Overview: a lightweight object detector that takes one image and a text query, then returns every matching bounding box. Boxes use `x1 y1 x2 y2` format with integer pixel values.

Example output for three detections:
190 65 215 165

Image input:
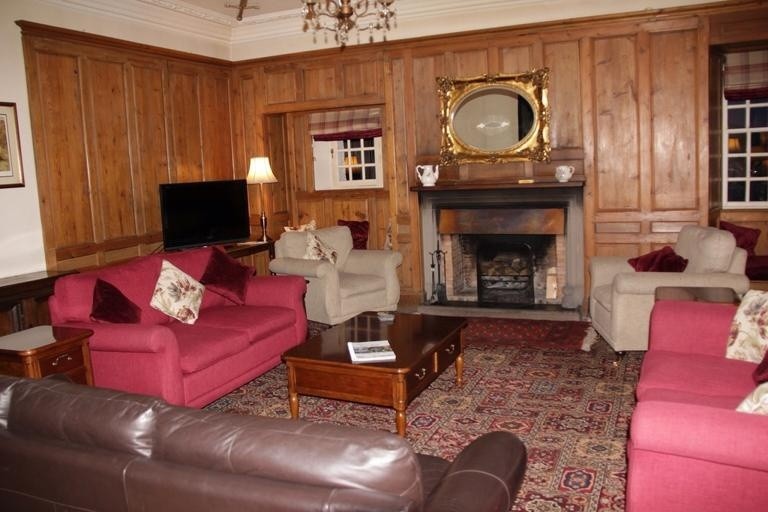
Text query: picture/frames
0 102 25 189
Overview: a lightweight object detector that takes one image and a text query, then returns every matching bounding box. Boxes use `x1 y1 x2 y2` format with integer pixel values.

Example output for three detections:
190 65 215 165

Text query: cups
554 166 576 182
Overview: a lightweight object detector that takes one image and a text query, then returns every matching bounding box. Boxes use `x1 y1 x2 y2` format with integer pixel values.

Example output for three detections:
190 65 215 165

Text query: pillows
54 245 256 324
628 220 762 272
279 219 369 267
726 289 768 416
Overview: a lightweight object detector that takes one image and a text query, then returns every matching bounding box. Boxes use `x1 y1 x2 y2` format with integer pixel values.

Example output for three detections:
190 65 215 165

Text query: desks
0 271 80 332
164 240 276 276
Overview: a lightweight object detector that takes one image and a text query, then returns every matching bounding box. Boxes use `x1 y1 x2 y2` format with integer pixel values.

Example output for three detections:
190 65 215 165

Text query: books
347 340 397 365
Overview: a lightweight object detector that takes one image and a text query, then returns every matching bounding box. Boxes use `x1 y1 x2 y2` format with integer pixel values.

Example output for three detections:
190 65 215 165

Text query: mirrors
435 66 552 166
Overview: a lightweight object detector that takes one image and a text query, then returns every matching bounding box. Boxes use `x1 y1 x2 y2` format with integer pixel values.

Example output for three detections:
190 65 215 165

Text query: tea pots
416 163 440 187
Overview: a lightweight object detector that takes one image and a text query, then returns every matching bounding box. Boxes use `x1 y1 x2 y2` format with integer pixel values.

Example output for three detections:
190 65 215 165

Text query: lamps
300 0 397 50
246 157 278 242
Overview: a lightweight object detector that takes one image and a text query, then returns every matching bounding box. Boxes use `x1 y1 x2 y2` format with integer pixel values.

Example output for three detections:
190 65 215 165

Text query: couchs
0 373 526 512
48 244 309 408
626 301 768 512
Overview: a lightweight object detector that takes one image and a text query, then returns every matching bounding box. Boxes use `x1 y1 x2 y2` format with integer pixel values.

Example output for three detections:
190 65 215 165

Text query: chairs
268 226 403 326
588 225 750 359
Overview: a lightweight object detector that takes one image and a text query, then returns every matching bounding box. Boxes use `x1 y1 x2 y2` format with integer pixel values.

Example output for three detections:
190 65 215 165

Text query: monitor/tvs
159 178 251 251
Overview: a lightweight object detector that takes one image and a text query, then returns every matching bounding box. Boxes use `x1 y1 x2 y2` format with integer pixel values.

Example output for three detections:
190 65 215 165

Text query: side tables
0 325 96 387
655 286 741 304
